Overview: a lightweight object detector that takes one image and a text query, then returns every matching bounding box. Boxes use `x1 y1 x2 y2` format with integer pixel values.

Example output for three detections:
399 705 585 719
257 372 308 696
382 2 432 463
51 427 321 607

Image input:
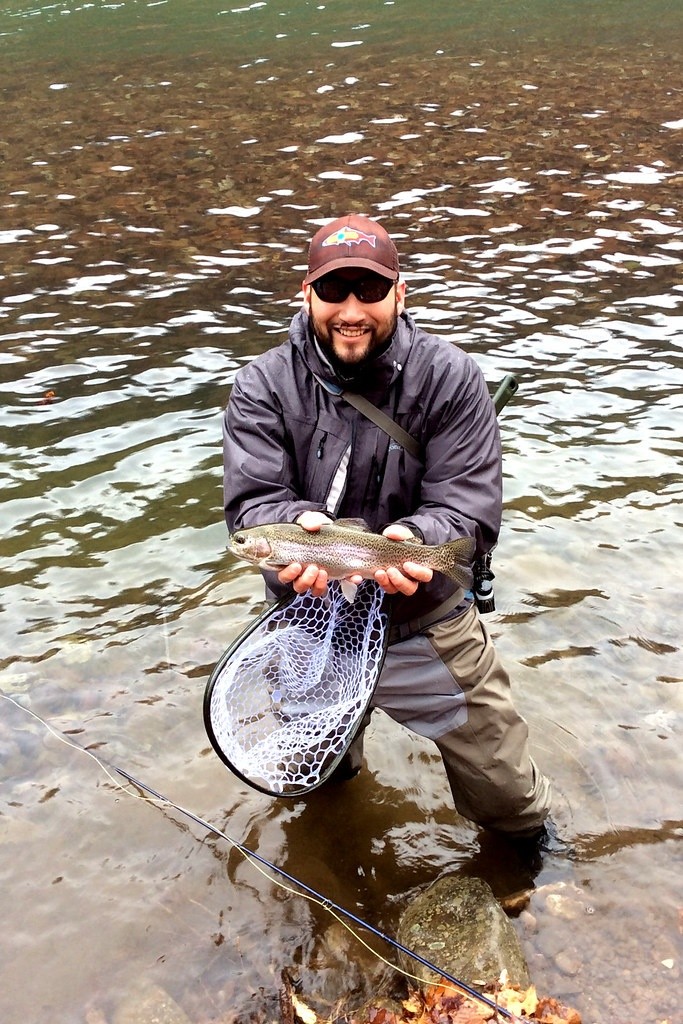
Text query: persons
224 213 575 887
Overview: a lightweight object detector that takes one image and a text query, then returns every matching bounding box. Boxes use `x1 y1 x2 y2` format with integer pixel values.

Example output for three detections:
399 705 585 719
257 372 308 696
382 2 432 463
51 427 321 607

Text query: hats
304 214 399 287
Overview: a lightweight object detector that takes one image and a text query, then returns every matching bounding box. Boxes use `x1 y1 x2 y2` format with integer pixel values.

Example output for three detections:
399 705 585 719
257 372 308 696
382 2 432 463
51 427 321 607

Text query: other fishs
222 517 479 602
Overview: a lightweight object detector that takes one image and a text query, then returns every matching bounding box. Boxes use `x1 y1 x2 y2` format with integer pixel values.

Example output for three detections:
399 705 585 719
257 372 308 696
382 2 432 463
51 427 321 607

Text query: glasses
311 277 394 304
